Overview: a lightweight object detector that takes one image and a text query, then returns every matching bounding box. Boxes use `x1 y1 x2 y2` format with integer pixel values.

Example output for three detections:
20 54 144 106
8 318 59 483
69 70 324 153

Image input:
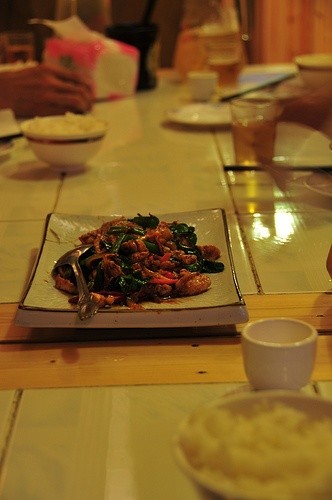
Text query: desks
0 64 332 500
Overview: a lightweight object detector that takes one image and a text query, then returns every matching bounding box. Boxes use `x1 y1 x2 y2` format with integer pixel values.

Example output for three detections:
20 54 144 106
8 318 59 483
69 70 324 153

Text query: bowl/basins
21 115 109 171
171 389 332 500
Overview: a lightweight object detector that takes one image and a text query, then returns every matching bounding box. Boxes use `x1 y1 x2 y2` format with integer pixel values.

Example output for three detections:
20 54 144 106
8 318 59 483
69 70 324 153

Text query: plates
12 206 250 327
266 120 332 169
167 102 248 127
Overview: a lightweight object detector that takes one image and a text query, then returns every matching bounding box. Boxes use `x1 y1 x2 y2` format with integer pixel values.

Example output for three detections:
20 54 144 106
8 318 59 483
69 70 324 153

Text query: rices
28 112 103 134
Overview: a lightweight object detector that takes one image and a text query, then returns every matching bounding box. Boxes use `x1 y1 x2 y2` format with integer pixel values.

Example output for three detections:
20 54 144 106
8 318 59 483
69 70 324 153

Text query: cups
241 317 318 391
294 53 332 83
187 70 218 102
230 97 278 185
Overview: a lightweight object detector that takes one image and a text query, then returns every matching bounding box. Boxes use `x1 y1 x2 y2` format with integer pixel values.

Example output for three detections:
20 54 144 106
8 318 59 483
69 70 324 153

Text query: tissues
26 13 141 102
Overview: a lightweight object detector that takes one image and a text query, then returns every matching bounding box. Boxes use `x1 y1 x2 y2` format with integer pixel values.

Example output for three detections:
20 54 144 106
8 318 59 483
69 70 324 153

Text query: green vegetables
51 212 225 296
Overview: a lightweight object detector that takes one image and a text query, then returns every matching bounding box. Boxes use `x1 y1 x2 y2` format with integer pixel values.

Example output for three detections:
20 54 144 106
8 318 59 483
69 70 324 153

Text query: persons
0 61 93 121
325 246 332 278
